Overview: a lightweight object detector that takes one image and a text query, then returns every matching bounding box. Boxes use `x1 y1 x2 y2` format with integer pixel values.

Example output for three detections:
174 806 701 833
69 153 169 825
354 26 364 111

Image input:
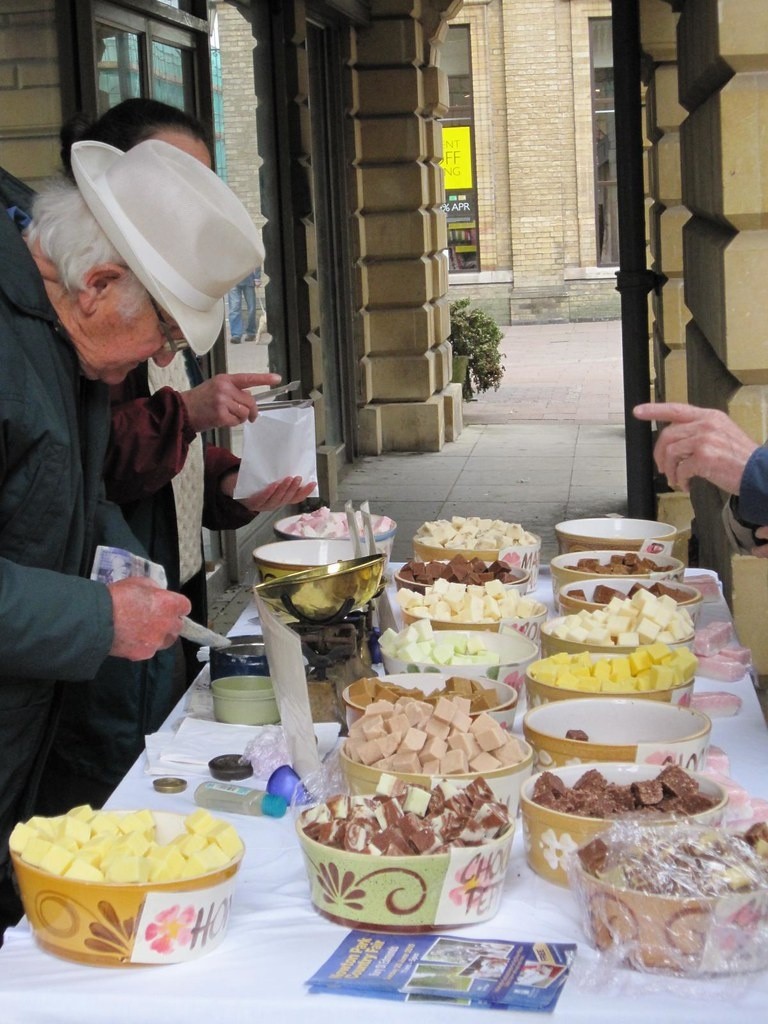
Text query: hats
71 139 266 355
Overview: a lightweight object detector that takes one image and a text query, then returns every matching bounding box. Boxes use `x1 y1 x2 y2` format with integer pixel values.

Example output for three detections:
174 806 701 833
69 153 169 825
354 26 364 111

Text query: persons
57 97 317 684
632 402 768 559
0 138 266 947
226 264 263 344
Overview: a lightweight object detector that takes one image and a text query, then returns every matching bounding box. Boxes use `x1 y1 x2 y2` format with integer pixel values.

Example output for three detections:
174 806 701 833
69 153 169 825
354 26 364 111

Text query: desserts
8 804 241 885
279 506 768 898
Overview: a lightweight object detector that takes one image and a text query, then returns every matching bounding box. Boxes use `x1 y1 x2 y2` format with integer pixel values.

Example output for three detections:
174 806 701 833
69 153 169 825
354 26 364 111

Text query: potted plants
449 298 507 404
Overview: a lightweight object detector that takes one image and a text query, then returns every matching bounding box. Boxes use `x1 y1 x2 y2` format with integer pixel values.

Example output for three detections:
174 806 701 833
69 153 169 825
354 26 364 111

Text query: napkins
144 715 342 776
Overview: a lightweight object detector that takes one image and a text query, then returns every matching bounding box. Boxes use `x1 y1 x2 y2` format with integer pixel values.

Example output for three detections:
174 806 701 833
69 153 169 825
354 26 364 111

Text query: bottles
195 781 287 819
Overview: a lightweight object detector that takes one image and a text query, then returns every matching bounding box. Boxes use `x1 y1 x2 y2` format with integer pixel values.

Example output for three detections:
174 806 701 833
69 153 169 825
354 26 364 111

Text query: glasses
148 288 189 353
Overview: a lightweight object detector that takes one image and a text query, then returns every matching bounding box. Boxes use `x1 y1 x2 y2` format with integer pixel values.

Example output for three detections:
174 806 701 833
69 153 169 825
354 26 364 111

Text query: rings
234 404 241 417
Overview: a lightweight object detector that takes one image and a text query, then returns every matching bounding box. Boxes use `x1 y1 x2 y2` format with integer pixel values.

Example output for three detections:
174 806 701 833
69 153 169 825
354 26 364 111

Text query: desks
0 563 768 1024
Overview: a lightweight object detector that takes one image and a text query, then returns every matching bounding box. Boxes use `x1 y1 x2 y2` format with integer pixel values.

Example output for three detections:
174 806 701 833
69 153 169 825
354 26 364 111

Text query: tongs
253 381 312 411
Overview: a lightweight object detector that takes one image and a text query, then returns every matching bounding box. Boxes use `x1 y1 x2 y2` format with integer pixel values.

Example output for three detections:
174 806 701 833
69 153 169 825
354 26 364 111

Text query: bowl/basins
555 518 677 556
579 836 768 977
210 635 269 680
341 736 533 831
380 630 539 693
343 674 517 731
520 762 730 888
273 512 397 562
252 539 385 582
541 613 695 660
525 652 695 708
413 532 541 592
296 794 515 934
394 560 530 595
9 812 245 964
523 697 711 768
254 554 388 620
211 676 280 724
550 550 703 628
402 600 549 646
